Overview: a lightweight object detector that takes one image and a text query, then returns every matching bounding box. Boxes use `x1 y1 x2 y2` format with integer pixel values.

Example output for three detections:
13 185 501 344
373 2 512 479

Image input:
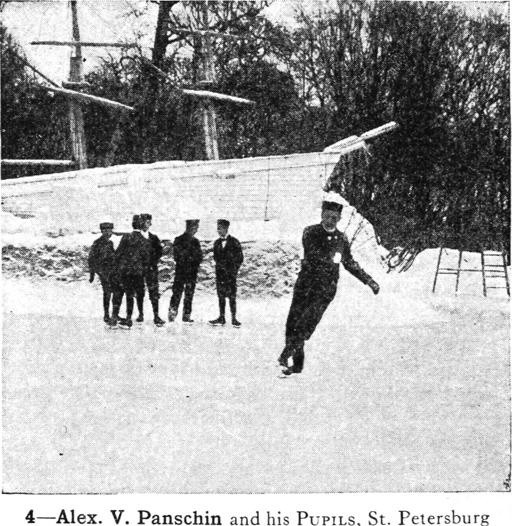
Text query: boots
279 352 304 374
103 311 241 326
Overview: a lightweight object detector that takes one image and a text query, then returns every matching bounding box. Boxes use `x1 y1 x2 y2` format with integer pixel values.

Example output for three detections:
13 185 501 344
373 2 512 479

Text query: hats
98 213 233 233
322 201 343 212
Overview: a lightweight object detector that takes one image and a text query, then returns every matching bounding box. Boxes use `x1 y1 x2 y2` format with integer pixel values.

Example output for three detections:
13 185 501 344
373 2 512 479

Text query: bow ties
222 239 227 241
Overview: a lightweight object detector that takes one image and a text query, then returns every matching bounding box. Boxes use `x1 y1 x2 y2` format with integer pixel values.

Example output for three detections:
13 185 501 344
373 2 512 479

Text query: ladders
431 169 510 301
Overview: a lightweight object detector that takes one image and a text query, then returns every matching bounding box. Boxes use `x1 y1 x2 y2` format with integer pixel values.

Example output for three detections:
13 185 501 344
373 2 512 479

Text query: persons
209 219 243 328
108 215 152 326
169 220 203 322
135 212 164 326
89 222 116 324
271 202 380 379
